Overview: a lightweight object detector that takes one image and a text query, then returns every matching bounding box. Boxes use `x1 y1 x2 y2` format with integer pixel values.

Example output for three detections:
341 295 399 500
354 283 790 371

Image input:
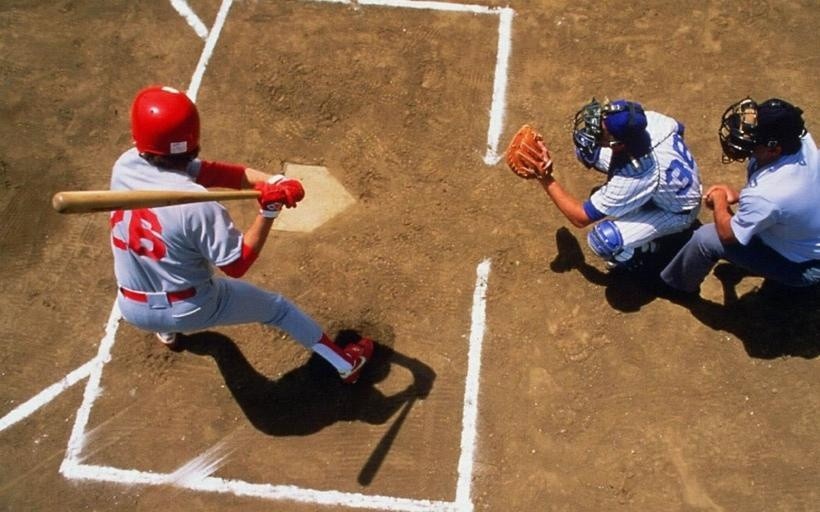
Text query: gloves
254 171 308 219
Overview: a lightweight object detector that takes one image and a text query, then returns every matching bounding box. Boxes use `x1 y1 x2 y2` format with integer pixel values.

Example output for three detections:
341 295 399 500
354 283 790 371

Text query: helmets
572 98 647 169
129 84 202 162
716 96 808 166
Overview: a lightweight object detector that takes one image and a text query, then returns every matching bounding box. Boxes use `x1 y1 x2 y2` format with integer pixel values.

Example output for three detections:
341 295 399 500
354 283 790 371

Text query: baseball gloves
507 124 553 179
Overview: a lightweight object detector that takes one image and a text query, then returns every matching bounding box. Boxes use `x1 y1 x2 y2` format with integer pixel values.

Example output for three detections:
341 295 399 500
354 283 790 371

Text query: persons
504 92 703 288
103 84 377 387
639 96 820 311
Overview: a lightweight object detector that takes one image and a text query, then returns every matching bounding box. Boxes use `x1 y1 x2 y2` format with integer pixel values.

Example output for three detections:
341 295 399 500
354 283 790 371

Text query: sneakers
156 328 178 349
620 236 702 301
339 334 377 388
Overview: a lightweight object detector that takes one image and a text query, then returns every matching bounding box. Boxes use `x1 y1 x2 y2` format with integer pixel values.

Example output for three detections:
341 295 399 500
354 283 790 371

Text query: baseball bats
53 189 262 214
